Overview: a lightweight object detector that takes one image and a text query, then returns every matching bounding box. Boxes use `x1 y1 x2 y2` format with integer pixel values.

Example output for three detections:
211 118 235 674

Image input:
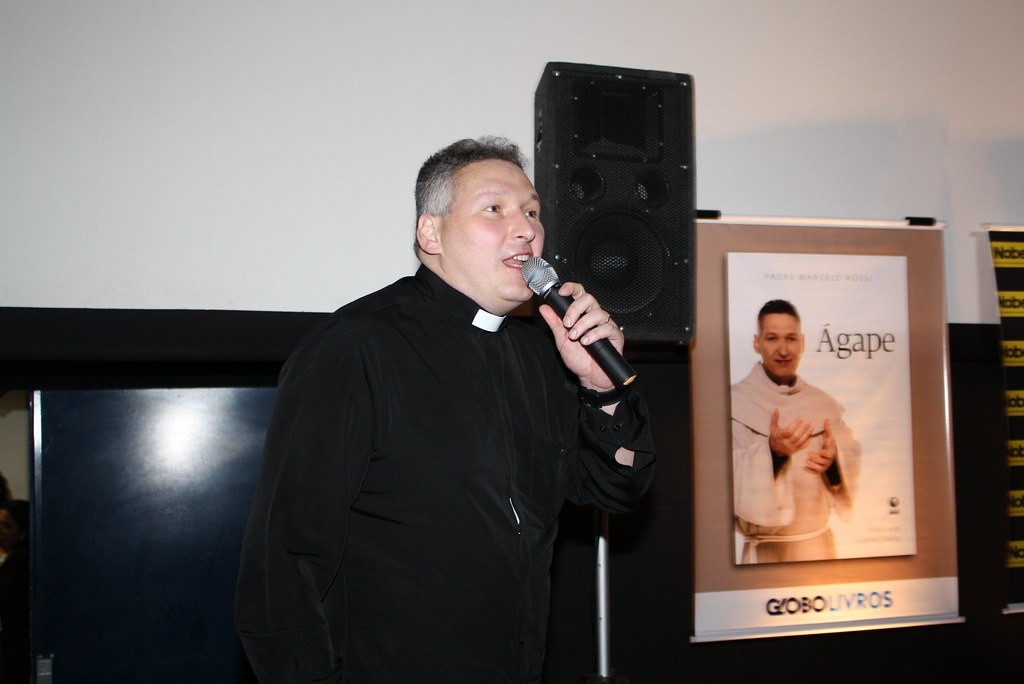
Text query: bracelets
579 385 619 410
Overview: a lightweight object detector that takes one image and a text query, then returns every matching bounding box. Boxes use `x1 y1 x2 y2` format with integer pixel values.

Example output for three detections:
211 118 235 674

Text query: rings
604 313 610 323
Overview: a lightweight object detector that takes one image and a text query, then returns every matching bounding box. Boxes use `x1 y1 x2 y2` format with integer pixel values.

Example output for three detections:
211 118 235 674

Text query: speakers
532 61 695 342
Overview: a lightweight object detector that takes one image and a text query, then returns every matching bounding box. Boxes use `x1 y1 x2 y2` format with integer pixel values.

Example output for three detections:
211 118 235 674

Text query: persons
730 300 864 562
233 137 654 684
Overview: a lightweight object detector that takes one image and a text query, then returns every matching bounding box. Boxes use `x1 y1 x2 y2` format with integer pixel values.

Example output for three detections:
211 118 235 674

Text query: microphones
520 257 638 388
